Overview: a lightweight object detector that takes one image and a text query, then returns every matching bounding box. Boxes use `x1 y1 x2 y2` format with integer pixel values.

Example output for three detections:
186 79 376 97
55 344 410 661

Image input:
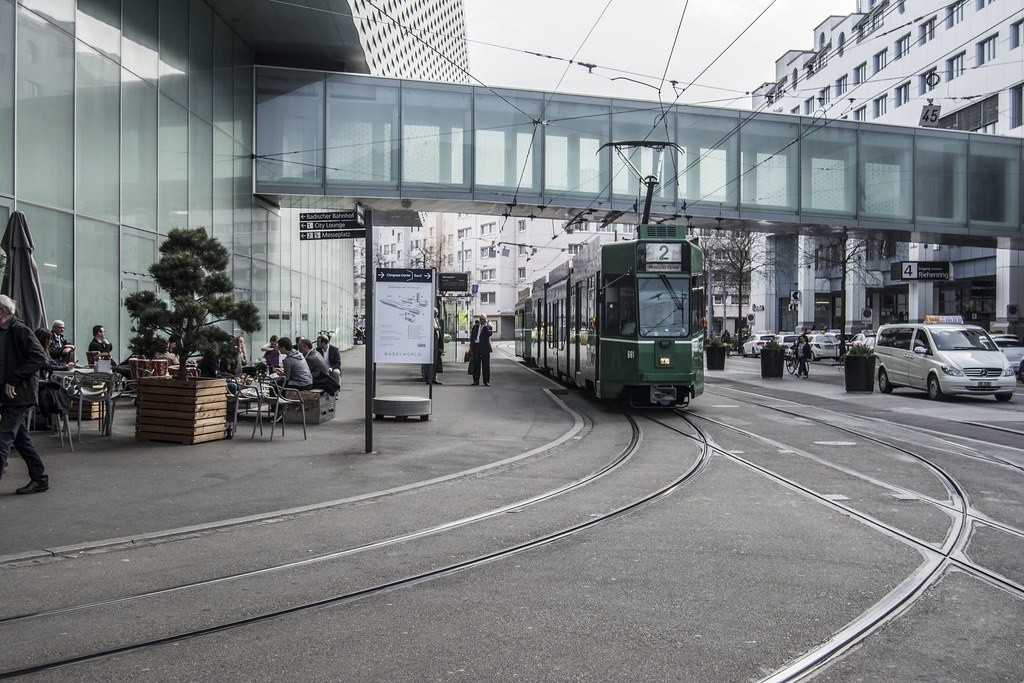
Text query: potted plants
836 342 880 394
122 227 262 445
760 337 787 378
705 337 727 370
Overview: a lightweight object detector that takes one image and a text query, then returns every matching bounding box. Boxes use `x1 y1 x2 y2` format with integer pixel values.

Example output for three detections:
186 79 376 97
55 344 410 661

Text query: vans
872 315 1018 400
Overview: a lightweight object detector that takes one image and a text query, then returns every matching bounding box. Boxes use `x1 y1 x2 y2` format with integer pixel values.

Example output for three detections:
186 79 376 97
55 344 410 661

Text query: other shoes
270 410 283 423
471 382 479 385
795 373 800 378
803 376 808 378
484 382 490 386
47 425 65 432
432 380 442 384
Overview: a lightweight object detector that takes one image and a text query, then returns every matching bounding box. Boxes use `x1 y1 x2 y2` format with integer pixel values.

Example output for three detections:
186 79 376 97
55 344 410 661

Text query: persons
794 337 811 378
261 335 282 366
314 335 341 385
721 330 730 344
165 341 179 364
470 314 493 386
88 325 133 391
0 295 49 494
298 339 329 376
421 308 444 385
34 328 74 430
270 337 313 422
48 320 74 365
650 305 667 323
197 337 267 413
293 336 304 351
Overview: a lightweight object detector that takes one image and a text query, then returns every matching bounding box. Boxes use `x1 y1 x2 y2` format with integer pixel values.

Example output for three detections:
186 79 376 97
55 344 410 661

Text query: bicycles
784 346 810 377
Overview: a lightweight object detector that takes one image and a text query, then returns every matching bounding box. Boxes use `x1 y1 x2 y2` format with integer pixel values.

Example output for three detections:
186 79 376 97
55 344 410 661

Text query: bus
510 137 718 411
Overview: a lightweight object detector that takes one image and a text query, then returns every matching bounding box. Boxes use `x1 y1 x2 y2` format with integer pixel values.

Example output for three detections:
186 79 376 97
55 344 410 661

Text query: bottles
266 366 270 376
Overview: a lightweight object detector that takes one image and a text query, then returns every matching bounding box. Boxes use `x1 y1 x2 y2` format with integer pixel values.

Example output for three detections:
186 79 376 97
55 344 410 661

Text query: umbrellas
0 211 49 332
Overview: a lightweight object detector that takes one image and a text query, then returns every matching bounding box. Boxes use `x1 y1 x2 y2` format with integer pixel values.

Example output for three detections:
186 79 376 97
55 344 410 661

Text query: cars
743 332 777 359
771 329 878 362
977 334 1024 382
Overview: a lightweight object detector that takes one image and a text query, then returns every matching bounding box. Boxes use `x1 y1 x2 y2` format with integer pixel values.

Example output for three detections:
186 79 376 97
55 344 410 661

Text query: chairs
188 366 307 443
22 367 155 452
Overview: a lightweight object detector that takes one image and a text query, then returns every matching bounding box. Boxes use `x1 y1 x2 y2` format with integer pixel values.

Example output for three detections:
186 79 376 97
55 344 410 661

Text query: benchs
284 389 338 425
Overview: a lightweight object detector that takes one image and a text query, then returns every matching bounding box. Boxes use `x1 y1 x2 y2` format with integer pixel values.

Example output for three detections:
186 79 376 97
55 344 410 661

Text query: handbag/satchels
316 371 340 396
468 358 474 375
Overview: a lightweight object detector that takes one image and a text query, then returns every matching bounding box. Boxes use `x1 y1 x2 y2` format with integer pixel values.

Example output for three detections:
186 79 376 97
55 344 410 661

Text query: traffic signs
300 211 359 220
298 229 367 240
299 220 366 232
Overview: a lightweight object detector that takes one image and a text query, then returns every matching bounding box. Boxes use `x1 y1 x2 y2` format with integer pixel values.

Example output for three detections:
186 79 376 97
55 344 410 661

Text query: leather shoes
16 480 49 494
